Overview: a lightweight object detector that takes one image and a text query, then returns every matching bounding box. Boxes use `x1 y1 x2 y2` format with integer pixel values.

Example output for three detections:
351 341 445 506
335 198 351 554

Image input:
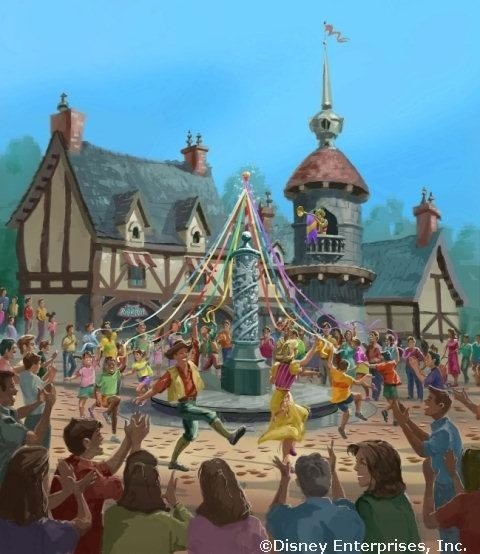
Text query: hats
163 335 194 359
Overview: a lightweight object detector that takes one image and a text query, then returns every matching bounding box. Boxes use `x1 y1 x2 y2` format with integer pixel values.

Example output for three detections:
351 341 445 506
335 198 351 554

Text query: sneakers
103 412 111 425
229 425 246 445
338 426 347 439
111 435 120 443
355 412 368 421
382 410 389 423
169 462 189 471
